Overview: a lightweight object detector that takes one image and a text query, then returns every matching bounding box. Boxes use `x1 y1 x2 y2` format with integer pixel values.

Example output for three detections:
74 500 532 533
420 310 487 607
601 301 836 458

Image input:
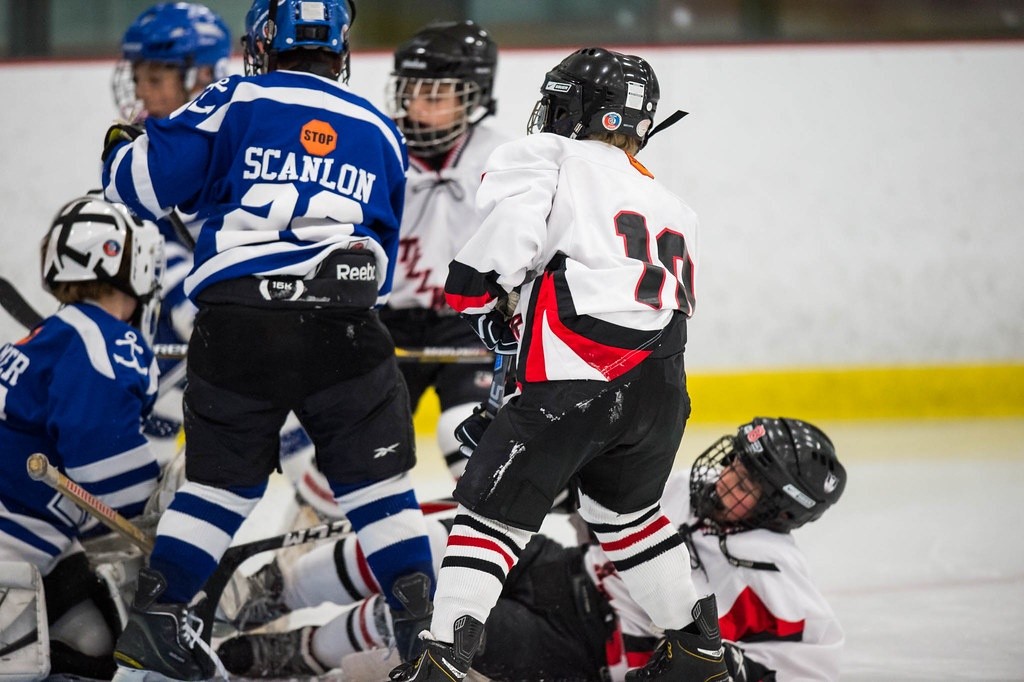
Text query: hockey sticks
187 518 356 682
0 277 497 367
26 451 157 555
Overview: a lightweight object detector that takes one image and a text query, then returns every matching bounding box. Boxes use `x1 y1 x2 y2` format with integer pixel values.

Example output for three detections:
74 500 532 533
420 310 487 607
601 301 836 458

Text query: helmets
240 0 356 86
40 196 168 300
690 416 847 535
527 47 660 150
111 3 232 125
385 20 496 158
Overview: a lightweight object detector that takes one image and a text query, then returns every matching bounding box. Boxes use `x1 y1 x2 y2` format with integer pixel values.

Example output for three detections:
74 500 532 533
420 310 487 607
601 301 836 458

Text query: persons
0 0 852 682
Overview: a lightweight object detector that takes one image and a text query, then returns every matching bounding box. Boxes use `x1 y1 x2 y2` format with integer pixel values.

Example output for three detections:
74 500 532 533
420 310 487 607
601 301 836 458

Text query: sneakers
114 567 216 682
387 615 485 682
624 593 730 682
216 626 327 679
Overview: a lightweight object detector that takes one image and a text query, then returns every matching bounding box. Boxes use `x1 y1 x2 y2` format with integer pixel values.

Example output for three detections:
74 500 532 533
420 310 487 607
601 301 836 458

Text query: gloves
459 305 522 355
99 125 144 162
